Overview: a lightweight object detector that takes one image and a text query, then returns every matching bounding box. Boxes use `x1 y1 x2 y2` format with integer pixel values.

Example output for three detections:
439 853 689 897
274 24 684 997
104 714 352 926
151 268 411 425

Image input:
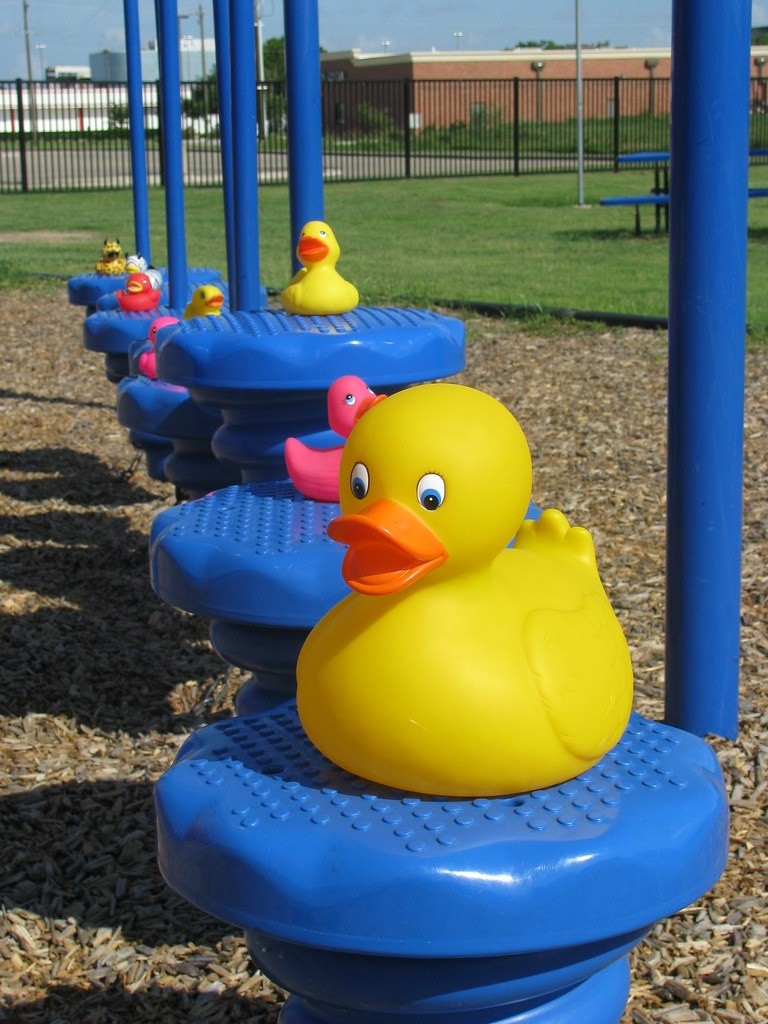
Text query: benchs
600 187 768 239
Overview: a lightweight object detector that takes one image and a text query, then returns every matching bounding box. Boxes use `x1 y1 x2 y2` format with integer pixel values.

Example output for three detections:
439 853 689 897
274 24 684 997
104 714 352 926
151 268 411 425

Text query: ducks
285 376 633 797
280 221 359 315
116 273 224 382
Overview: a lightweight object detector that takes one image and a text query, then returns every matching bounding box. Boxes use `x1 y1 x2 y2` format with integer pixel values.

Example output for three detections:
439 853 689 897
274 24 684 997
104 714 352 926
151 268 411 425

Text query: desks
616 149 768 234
